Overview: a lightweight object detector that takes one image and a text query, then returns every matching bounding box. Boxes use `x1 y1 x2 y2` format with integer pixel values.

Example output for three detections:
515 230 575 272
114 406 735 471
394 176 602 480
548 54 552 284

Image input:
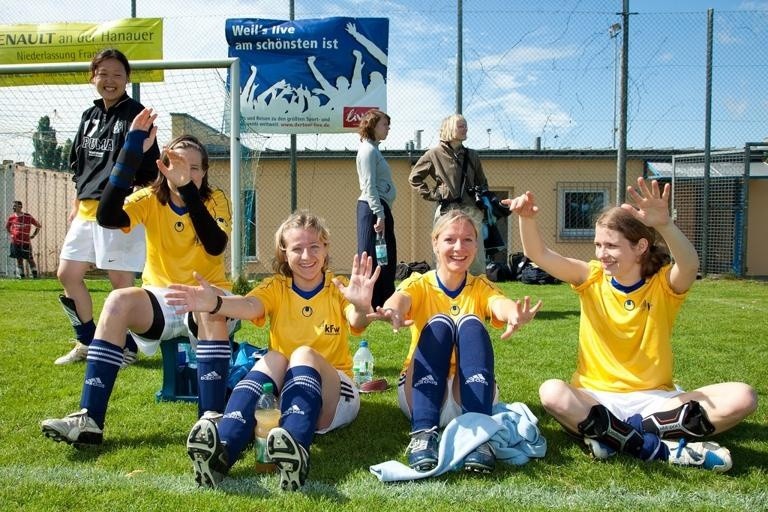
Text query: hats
359 379 392 393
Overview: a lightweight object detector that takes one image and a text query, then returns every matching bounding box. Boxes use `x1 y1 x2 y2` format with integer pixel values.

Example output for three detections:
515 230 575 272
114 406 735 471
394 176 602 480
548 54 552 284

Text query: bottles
254 383 282 474
352 341 374 392
374 230 388 266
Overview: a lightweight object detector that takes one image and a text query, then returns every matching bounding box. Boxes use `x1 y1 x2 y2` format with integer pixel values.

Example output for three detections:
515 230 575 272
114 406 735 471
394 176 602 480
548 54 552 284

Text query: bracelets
208 295 223 315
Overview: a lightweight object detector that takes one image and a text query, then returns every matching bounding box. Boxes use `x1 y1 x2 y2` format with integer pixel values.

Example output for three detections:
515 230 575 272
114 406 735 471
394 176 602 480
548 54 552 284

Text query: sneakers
660 439 732 473
41 408 104 450
463 440 496 473
266 428 309 491
186 418 231 491
403 426 441 472
122 348 137 366
584 414 642 459
54 340 90 365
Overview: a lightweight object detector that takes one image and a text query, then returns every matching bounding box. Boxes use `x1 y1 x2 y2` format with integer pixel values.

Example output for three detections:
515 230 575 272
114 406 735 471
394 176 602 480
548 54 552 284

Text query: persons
363 208 543 478
30 114 74 172
38 105 234 455
407 114 496 281
498 174 759 475
163 208 383 496
236 21 386 117
51 47 164 367
356 109 398 314
4 200 42 279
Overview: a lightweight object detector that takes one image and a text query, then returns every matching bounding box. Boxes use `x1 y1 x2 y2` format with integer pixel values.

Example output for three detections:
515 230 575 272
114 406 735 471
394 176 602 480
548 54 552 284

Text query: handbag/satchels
395 261 431 280
486 252 561 284
481 196 504 248
